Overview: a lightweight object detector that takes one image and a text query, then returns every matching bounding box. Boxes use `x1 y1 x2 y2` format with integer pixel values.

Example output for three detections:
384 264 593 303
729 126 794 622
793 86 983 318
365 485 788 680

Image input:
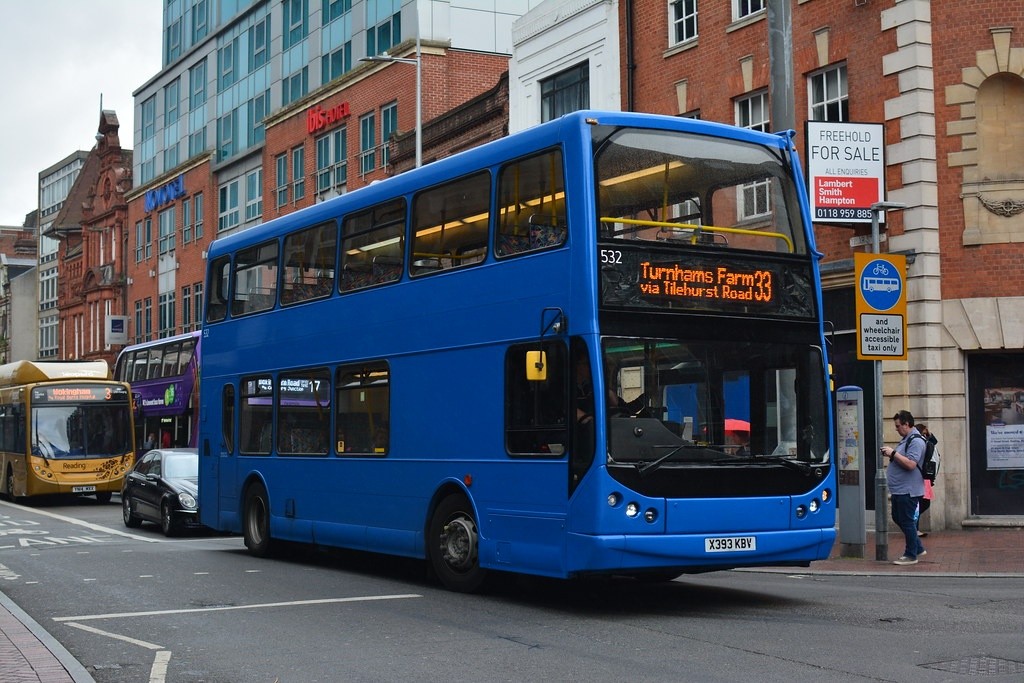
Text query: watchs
891 450 896 457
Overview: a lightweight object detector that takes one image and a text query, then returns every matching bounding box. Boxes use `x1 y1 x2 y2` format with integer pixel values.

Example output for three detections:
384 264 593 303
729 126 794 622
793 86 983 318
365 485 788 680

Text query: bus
115 327 202 459
199 109 838 590
0 358 135 504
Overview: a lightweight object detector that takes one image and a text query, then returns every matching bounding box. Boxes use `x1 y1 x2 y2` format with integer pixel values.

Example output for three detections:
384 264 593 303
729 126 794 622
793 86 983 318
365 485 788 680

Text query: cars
122 448 218 539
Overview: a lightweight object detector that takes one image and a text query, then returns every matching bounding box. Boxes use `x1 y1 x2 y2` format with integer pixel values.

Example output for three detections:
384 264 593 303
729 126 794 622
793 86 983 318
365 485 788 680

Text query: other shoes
917 533 928 537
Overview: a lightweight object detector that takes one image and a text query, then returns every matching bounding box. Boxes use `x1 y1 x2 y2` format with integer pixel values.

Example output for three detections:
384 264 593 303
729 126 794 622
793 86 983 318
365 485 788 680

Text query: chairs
106 213 595 386
250 404 392 457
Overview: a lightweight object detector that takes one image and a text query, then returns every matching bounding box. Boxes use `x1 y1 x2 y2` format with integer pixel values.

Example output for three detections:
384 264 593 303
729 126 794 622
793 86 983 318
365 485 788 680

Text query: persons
575 358 645 427
145 432 158 449
914 424 939 537
879 410 927 565
372 426 389 454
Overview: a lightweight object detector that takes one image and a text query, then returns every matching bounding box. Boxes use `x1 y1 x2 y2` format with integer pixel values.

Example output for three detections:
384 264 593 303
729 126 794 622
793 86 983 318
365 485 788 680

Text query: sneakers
918 550 926 557
892 555 918 564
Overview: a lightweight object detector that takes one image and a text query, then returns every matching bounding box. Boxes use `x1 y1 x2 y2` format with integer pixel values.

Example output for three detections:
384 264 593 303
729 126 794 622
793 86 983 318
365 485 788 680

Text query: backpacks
921 440 942 482
